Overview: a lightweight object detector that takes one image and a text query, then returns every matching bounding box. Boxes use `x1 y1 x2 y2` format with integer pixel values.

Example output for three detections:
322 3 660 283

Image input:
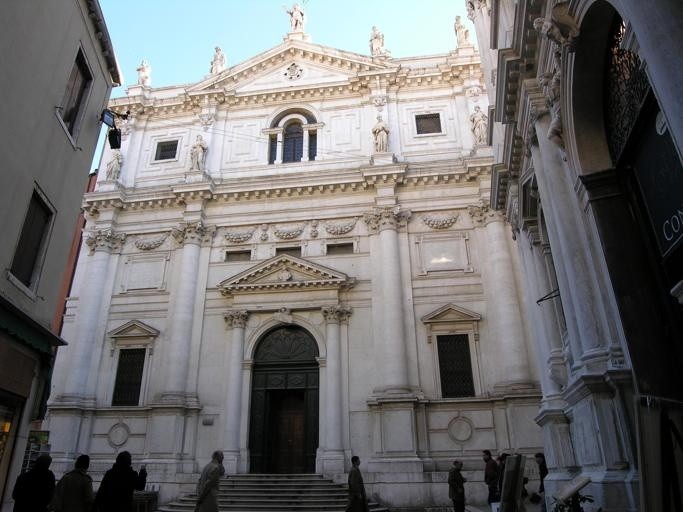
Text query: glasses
102 105 122 149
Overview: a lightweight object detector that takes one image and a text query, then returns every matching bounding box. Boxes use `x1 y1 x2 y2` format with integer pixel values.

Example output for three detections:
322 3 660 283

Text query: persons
10 452 55 511
481 449 500 511
452 16 473 47
469 104 489 146
48 453 92 512
187 134 208 171
369 114 391 154
496 452 511 492
90 450 146 511
282 3 304 32
209 46 226 75
368 26 385 56
534 452 548 493
446 459 467 512
344 455 369 512
535 68 569 162
191 448 224 511
532 17 567 47
104 147 123 181
135 58 150 87
